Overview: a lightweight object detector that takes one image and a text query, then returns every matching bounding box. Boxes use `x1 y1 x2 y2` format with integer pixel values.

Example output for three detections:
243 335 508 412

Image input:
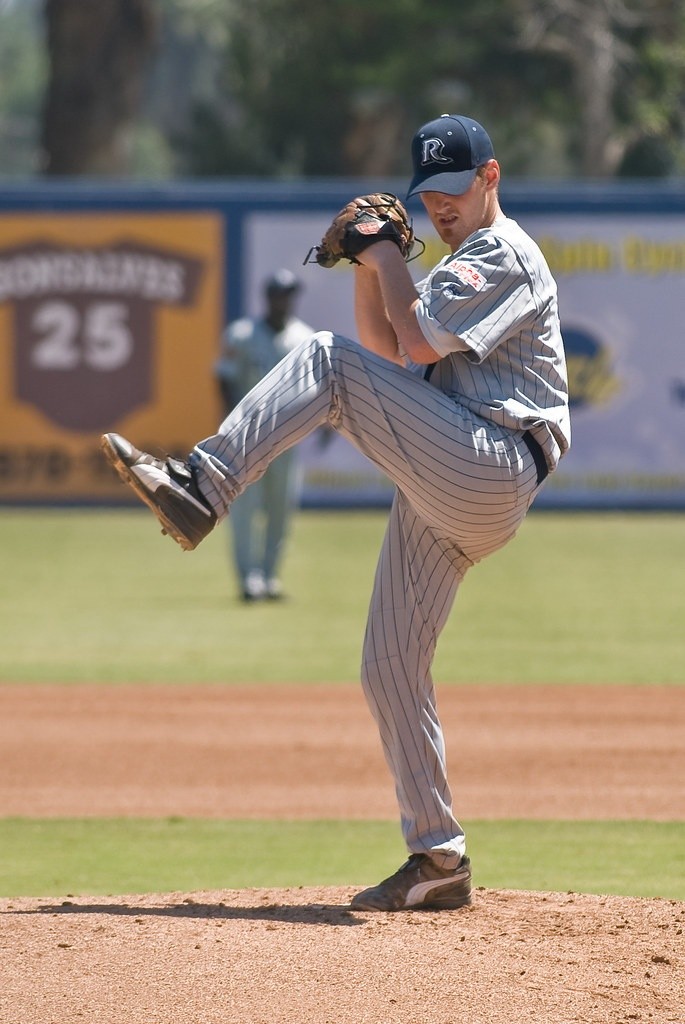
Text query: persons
216 269 317 603
101 114 572 908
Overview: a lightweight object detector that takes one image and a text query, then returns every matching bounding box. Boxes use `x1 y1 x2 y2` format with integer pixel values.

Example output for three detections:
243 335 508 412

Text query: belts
522 430 550 485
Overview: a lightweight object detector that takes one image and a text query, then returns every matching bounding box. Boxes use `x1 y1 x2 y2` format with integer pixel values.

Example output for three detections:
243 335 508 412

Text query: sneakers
101 432 217 551
349 853 472 911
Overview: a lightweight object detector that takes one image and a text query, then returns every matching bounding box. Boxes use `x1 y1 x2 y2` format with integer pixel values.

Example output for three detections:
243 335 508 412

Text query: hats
406 113 494 201
267 273 305 296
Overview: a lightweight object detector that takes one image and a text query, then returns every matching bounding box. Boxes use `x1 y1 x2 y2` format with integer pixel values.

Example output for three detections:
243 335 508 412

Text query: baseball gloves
300 192 427 270
